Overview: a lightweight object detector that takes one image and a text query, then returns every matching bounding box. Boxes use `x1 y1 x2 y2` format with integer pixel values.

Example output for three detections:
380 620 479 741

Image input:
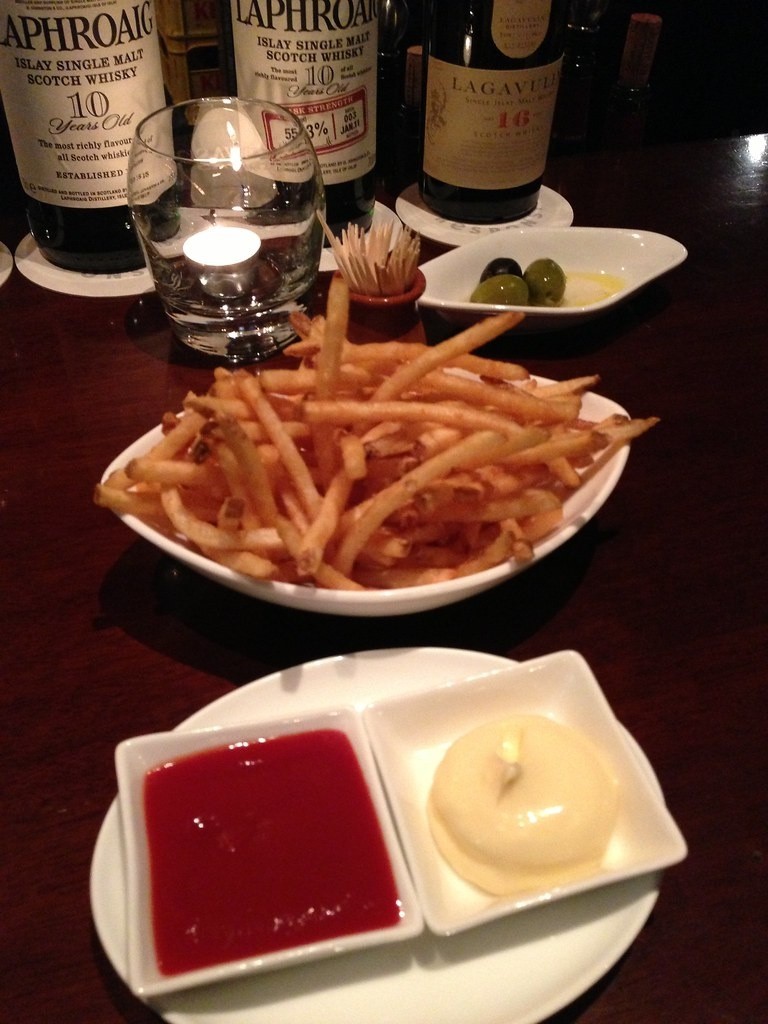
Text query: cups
126 96 325 360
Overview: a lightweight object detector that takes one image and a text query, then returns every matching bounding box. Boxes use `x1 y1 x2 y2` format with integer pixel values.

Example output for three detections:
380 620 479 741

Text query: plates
421 226 688 324
99 368 631 618
90 648 659 1024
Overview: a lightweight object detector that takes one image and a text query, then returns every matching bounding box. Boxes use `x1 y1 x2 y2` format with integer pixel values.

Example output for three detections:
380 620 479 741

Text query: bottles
377 0 667 224
331 267 428 344
222 0 378 248
0 0 180 276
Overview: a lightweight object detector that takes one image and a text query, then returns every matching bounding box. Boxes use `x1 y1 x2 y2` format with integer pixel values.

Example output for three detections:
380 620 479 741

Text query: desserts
426 714 616 899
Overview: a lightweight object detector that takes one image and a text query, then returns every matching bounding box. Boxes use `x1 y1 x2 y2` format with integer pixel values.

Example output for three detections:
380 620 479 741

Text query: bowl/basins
364 649 688 938
114 707 424 998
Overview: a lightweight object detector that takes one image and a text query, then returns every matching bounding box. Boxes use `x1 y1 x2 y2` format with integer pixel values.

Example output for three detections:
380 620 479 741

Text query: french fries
94 280 663 591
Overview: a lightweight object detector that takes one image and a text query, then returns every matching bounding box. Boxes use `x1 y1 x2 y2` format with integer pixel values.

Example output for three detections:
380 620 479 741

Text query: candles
183 207 261 299
